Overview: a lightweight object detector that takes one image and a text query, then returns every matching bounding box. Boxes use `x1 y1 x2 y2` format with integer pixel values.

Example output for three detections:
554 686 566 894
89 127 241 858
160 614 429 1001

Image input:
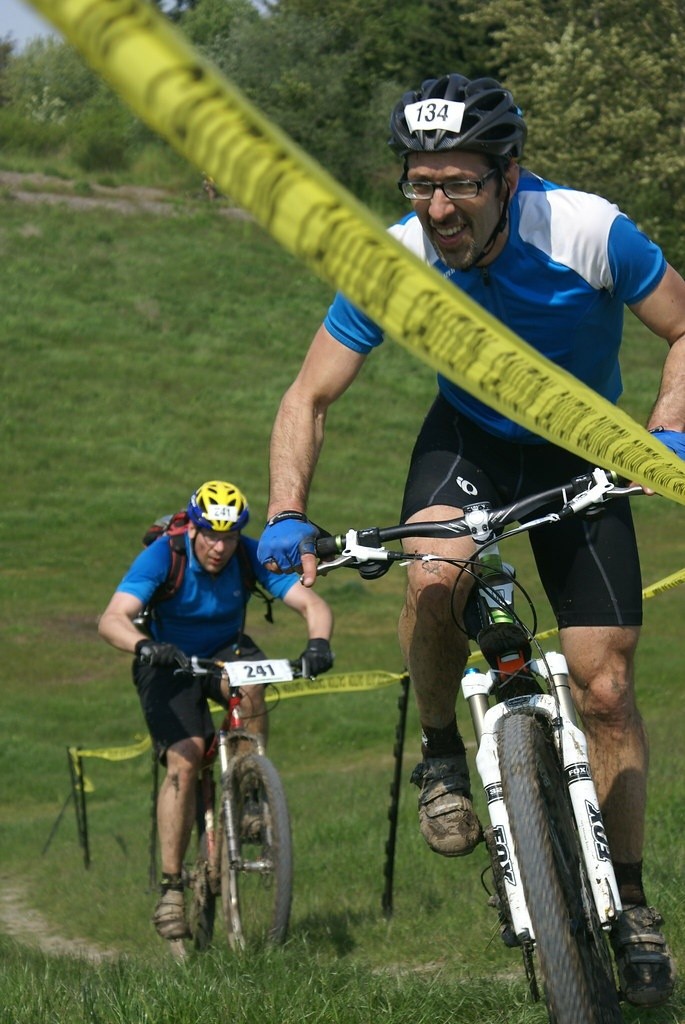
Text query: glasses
396 161 499 200
201 531 243 546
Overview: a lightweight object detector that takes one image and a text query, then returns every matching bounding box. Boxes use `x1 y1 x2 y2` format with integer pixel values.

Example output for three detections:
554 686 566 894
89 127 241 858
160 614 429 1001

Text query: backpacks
141 508 190 600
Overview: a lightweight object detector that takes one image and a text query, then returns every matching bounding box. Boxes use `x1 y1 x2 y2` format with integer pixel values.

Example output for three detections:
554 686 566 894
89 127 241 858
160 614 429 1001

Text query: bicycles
297 427 665 1023
135 651 337 967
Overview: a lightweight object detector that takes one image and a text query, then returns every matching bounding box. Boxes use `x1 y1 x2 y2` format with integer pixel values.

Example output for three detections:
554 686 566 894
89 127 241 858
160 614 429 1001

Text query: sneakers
410 752 482 859
607 898 676 1008
150 886 190 942
239 813 265 841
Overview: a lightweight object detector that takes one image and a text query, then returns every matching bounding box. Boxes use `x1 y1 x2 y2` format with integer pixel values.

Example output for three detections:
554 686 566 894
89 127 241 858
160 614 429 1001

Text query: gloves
288 638 334 682
135 639 190 671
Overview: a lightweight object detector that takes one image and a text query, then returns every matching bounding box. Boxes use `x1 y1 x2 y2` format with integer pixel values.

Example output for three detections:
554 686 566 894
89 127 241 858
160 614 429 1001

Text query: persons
256 73 685 1007
93 480 338 941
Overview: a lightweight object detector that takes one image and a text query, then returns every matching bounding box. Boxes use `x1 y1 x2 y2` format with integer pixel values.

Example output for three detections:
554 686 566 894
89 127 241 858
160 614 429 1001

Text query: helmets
187 480 250 533
387 72 527 162
